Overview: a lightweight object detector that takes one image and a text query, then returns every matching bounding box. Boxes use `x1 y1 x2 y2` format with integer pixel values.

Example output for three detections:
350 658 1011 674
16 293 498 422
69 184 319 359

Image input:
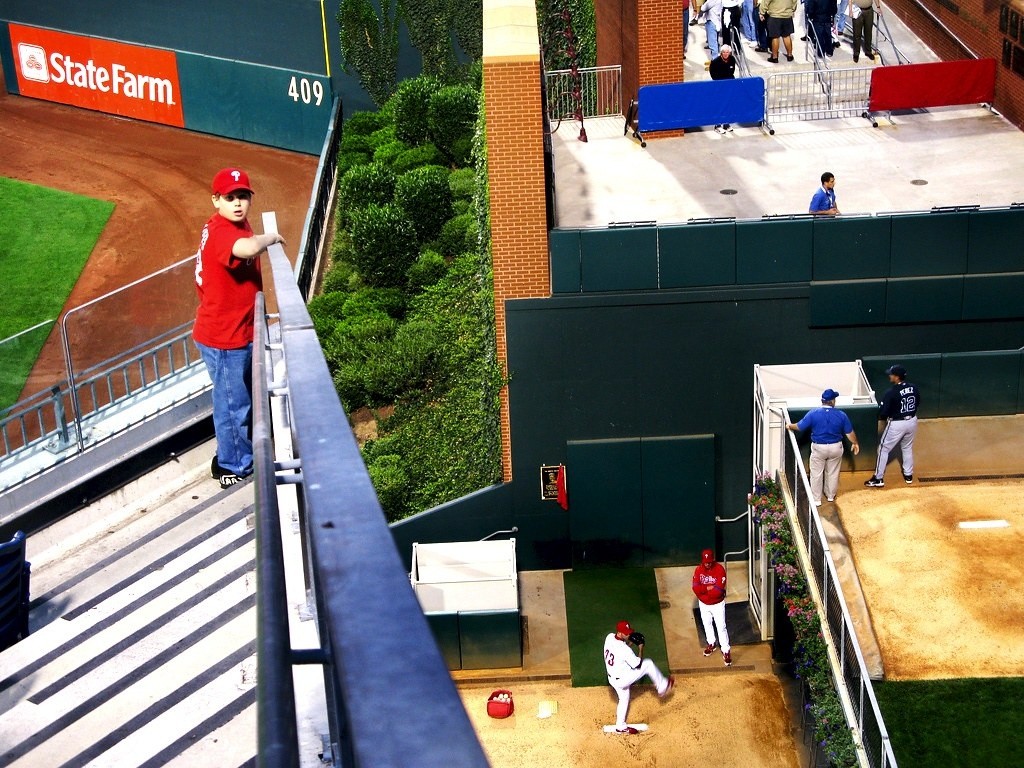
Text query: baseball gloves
628 632 645 647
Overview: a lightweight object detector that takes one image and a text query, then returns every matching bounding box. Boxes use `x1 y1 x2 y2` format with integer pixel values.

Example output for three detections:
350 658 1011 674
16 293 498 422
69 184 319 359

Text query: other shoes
749 41 758 48
853 54 859 62
211 455 231 479
755 47 769 52
815 501 821 506
723 125 733 132
767 55 779 63
714 125 726 135
220 467 253 490
787 55 794 61
828 497 834 501
865 52 875 60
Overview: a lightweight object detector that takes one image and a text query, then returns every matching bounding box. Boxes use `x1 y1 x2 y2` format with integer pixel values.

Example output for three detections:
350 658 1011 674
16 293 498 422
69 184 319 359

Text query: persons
809 172 840 215
786 390 859 506
682 0 797 71
801 0 849 58
192 167 287 489
865 365 921 487
604 622 675 735
693 550 731 666
709 44 736 134
849 0 883 62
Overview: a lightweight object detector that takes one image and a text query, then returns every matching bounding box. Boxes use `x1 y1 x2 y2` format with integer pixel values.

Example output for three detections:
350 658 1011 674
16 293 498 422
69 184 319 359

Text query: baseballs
493 693 510 703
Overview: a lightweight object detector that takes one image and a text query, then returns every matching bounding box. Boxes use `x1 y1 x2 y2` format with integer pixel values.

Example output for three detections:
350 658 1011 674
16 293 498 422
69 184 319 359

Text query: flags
555 463 568 510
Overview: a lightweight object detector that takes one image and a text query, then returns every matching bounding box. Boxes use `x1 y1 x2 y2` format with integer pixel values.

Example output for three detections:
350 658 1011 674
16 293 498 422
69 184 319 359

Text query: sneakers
703 642 718 656
660 678 675 697
905 475 913 483
616 727 638 734
864 475 884 486
721 650 732 666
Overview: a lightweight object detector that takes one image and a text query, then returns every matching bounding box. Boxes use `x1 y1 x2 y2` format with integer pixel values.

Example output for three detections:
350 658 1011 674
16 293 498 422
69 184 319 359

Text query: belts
891 413 915 421
812 439 842 445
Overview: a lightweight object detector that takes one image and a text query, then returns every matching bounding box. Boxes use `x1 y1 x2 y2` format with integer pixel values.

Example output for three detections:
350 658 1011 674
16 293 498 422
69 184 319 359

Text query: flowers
747 471 864 768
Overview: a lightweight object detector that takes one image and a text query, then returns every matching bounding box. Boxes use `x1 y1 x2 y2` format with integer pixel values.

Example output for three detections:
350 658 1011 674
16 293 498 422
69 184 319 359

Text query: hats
885 365 907 375
211 168 255 195
617 621 634 634
821 388 839 401
702 550 715 562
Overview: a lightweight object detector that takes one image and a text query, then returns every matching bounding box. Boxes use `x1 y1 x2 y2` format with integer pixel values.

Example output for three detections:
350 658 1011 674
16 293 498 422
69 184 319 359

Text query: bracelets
789 424 792 430
877 6 880 8
